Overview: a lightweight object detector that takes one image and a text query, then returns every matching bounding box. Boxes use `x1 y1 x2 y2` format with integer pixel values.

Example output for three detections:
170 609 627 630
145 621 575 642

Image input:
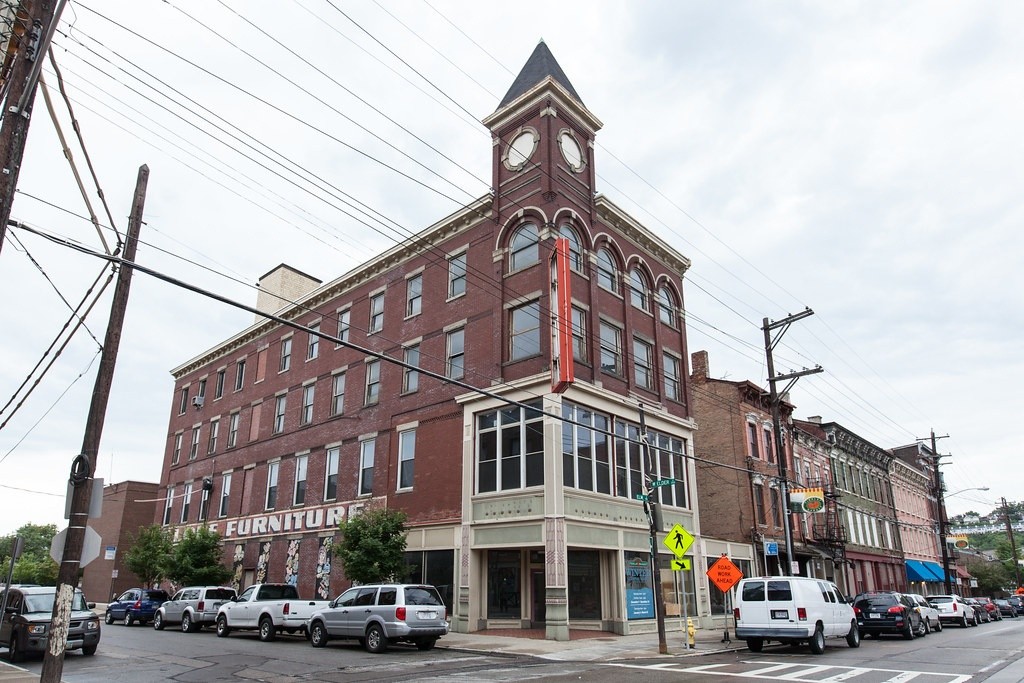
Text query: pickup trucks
214 582 331 641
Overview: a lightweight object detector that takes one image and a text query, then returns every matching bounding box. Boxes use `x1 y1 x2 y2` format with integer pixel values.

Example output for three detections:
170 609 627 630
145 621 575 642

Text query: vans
1011 594 1024 614
733 575 861 656
306 583 452 654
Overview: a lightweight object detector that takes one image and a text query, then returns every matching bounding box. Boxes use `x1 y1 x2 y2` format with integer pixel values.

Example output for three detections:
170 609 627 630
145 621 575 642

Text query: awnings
956 567 972 579
905 560 938 582
922 562 955 582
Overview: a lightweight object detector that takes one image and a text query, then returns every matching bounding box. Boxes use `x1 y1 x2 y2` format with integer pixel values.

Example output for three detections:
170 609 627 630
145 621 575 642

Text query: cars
105 586 170 626
854 590 927 640
993 598 1019 618
965 597 992 622
909 594 942 632
982 596 1004 620
929 592 979 628
153 584 238 633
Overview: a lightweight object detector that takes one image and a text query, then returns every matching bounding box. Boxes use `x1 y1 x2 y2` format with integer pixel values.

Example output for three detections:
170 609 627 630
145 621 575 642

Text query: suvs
0 584 100 662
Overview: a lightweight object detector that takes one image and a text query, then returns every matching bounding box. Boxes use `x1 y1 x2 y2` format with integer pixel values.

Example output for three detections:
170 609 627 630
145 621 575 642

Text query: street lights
941 485 990 501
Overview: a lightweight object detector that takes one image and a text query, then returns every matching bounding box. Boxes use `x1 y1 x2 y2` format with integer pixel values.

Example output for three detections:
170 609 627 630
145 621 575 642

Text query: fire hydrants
684 617 698 649
